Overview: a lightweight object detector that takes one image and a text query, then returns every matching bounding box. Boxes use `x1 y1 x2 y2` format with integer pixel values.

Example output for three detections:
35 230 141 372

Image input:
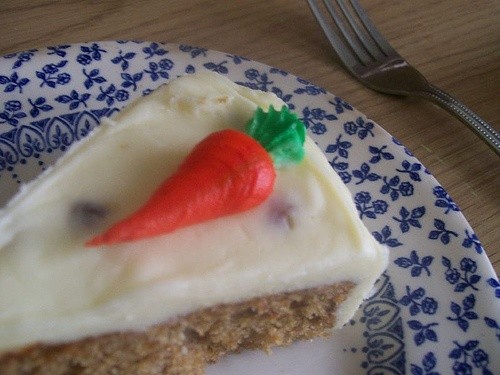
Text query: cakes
0 67 389 374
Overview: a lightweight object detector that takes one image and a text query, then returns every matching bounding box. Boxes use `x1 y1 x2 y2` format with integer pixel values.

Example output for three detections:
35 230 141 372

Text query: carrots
82 104 306 248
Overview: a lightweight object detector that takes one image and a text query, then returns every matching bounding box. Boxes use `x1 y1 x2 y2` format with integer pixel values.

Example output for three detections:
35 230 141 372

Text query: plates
1 40 500 375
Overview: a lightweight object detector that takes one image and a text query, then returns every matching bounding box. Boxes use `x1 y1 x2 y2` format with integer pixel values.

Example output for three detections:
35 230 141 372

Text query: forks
306 0 500 158
